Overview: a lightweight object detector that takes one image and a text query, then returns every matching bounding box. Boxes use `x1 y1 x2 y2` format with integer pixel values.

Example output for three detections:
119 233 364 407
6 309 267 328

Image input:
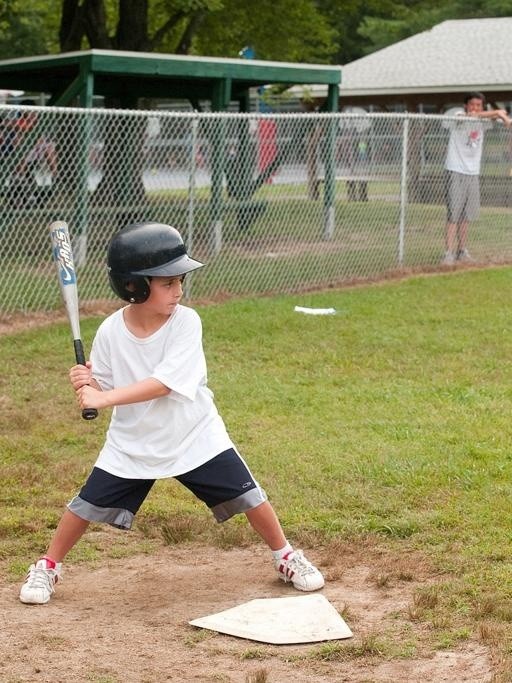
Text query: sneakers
456 249 478 264
18 556 60 606
441 252 455 266
275 549 326 593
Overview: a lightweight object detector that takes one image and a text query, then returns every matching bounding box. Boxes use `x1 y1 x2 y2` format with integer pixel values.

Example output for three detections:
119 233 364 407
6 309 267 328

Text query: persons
441 91 512 264
18 98 39 117
17 220 326 606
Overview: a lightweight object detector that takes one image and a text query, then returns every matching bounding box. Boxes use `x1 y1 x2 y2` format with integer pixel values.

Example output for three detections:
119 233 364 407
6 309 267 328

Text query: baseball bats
47 219 97 418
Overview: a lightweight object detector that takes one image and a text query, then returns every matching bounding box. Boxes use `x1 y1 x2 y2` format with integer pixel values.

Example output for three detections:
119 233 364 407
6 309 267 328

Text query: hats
106 220 207 305
